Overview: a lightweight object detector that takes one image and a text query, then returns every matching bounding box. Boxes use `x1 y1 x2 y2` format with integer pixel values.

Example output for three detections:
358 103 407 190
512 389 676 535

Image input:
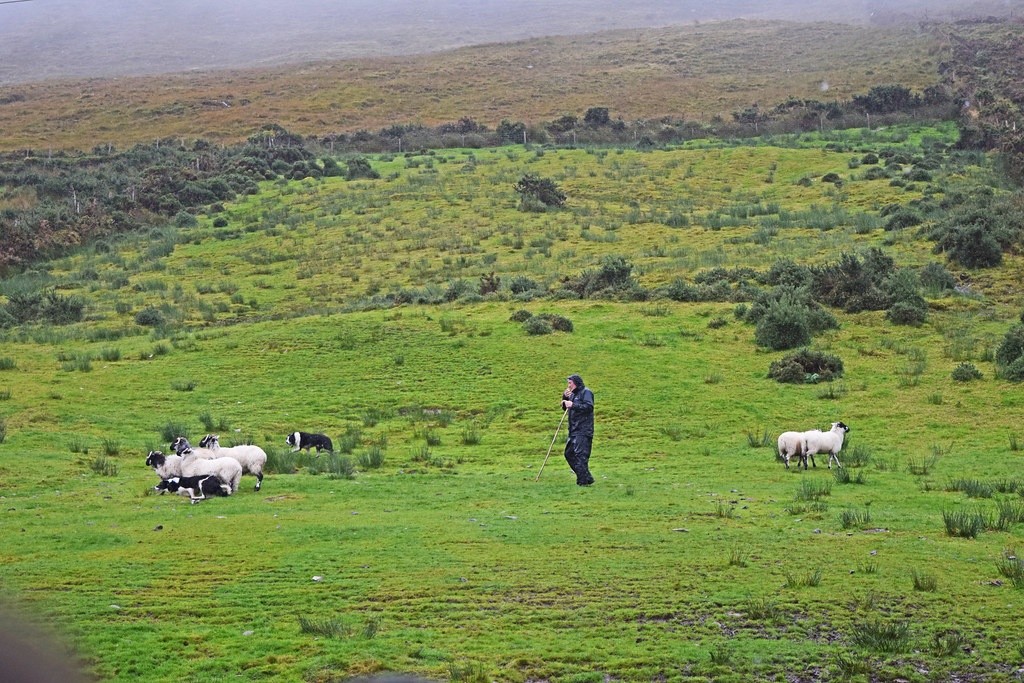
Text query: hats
567 375 583 387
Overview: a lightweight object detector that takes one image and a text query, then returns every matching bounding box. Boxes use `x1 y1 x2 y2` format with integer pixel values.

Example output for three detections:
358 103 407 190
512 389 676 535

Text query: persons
562 375 595 486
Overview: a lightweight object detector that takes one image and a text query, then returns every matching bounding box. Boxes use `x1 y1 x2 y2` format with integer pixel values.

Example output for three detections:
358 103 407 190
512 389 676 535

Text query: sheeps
145 434 268 496
777 421 850 469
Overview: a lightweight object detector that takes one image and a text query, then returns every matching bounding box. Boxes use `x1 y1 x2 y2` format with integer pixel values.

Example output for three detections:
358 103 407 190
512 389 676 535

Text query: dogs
286 432 334 460
153 474 233 506
